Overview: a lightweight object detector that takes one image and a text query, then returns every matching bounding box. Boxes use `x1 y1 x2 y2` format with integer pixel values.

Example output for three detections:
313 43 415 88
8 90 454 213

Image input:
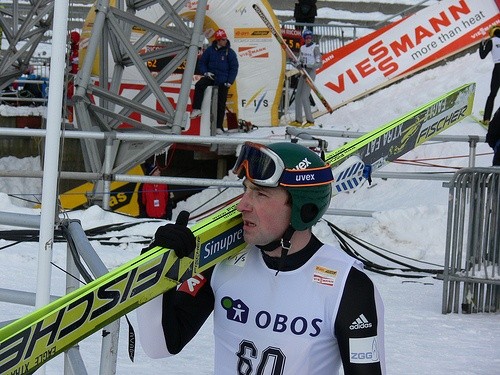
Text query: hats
214 29 226 40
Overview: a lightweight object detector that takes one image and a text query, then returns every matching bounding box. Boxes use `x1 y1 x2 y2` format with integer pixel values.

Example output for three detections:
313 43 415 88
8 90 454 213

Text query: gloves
155 210 198 259
224 83 231 87
204 72 216 81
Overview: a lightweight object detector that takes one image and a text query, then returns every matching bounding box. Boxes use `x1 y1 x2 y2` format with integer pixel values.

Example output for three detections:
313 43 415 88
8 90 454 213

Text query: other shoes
302 122 314 127
217 128 223 135
190 109 202 119
480 119 490 126
289 121 302 127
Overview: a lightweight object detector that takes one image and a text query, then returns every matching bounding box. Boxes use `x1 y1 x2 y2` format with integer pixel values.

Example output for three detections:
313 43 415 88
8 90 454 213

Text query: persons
287 30 323 128
66 30 80 112
141 166 169 219
188 28 239 134
478 26 500 122
293 0 317 34
485 107 500 166
123 140 388 375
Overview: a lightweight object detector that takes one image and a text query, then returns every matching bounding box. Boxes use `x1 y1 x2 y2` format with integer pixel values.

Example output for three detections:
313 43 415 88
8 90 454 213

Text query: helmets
489 26 500 37
24 64 34 74
265 142 333 230
302 30 313 38
69 32 80 43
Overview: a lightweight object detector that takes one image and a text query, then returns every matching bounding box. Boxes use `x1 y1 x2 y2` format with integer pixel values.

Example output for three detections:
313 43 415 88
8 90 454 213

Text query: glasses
221 39 226 41
232 141 335 188
305 37 312 40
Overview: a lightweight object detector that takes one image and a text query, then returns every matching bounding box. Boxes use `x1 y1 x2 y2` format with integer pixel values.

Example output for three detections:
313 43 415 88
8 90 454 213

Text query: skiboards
252 2 333 115
0 80 478 375
